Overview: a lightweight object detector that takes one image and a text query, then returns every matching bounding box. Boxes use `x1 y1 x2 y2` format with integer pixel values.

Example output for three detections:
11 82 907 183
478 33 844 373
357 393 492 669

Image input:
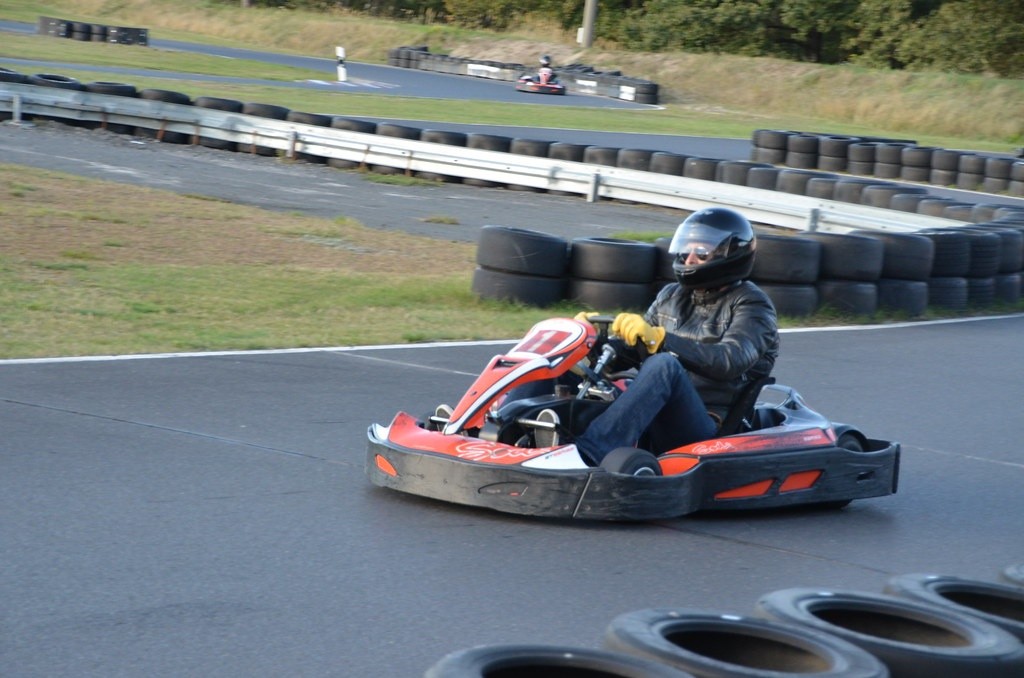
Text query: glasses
678 245 712 262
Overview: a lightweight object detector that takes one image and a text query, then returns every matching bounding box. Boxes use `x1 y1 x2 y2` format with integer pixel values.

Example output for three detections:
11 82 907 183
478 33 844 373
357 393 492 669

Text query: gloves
573 311 600 324
611 312 667 354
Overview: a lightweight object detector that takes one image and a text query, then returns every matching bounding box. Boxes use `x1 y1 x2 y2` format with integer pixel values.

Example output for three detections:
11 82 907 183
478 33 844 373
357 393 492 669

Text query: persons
531 56 557 84
425 206 780 467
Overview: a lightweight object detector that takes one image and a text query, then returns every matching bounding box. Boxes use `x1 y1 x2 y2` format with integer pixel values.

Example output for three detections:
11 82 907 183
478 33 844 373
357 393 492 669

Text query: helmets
538 55 551 66
667 206 757 293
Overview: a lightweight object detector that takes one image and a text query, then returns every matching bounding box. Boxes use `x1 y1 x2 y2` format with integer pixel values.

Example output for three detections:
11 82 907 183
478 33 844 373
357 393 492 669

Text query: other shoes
534 408 577 448
435 403 457 432
